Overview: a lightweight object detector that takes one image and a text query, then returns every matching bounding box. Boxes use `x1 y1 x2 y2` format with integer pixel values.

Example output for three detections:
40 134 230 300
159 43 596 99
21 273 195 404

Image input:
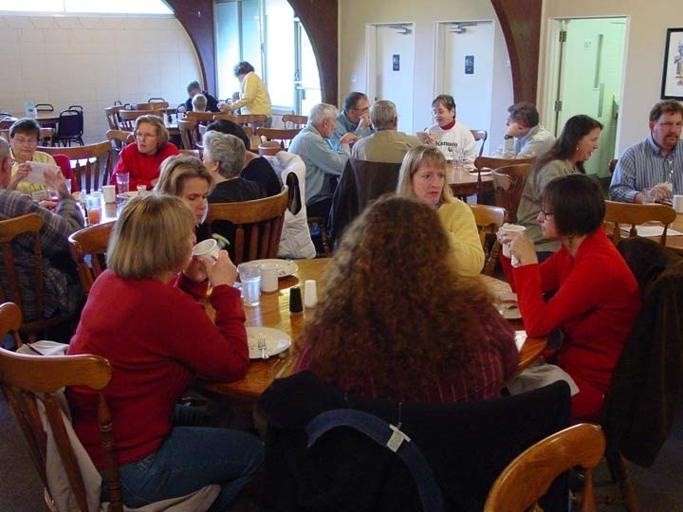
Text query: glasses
541 210 554 219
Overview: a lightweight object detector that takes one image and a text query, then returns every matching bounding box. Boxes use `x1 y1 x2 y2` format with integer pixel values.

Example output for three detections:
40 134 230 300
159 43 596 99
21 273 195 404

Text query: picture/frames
659 27 681 101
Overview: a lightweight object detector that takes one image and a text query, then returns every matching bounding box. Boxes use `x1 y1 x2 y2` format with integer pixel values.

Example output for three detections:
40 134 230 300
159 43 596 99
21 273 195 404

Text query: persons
0 62 683 511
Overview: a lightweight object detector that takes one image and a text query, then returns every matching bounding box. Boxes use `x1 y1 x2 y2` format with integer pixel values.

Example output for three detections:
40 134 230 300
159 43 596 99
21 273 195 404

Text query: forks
255 336 270 360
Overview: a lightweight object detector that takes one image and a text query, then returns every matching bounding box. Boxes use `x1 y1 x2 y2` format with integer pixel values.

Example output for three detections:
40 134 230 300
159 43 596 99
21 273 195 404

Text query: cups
170 114 176 125
136 184 146 195
102 185 115 204
236 261 261 306
80 185 102 224
32 108 37 114
450 147 464 171
672 195 683 215
177 113 183 121
641 187 656 206
116 171 129 195
260 264 278 293
63 179 71 193
498 224 526 258
452 168 462 183
191 237 223 268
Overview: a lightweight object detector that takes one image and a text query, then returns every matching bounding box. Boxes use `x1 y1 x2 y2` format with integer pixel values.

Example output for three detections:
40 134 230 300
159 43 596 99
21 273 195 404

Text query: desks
434 158 501 199
173 253 545 416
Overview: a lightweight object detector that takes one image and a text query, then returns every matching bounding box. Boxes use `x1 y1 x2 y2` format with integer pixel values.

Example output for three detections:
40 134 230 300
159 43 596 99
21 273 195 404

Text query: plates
243 325 294 361
490 291 523 320
237 258 299 277
116 192 148 199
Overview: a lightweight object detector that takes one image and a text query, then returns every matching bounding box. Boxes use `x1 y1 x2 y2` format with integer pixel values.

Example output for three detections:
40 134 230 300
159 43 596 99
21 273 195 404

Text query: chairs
488 161 538 279
1 210 56 333
468 126 488 161
0 99 343 188
0 299 125 510
475 153 537 196
589 188 681 259
481 419 608 510
586 196 674 293
191 180 292 262
65 216 116 296
453 200 510 276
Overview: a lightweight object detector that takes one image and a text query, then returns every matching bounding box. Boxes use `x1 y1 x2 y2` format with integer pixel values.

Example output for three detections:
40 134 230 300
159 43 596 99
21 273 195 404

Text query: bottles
288 286 303 315
303 279 317 309
